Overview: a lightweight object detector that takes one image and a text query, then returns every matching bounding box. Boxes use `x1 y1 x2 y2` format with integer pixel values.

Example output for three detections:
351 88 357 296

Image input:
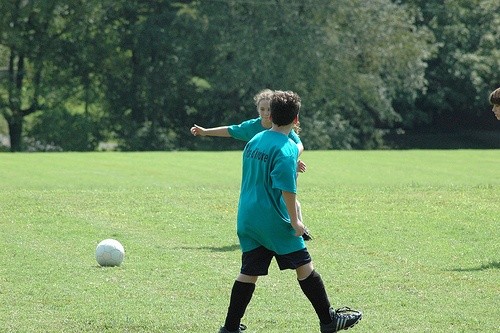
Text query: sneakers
320 312 361 333
217 325 242 333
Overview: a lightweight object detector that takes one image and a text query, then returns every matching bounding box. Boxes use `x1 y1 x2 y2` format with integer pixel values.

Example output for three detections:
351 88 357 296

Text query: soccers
96 239 125 266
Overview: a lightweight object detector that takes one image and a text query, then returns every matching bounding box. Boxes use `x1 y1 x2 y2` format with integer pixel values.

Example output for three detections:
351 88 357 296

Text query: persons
190 88 315 241
218 91 363 333
489 88 500 121
